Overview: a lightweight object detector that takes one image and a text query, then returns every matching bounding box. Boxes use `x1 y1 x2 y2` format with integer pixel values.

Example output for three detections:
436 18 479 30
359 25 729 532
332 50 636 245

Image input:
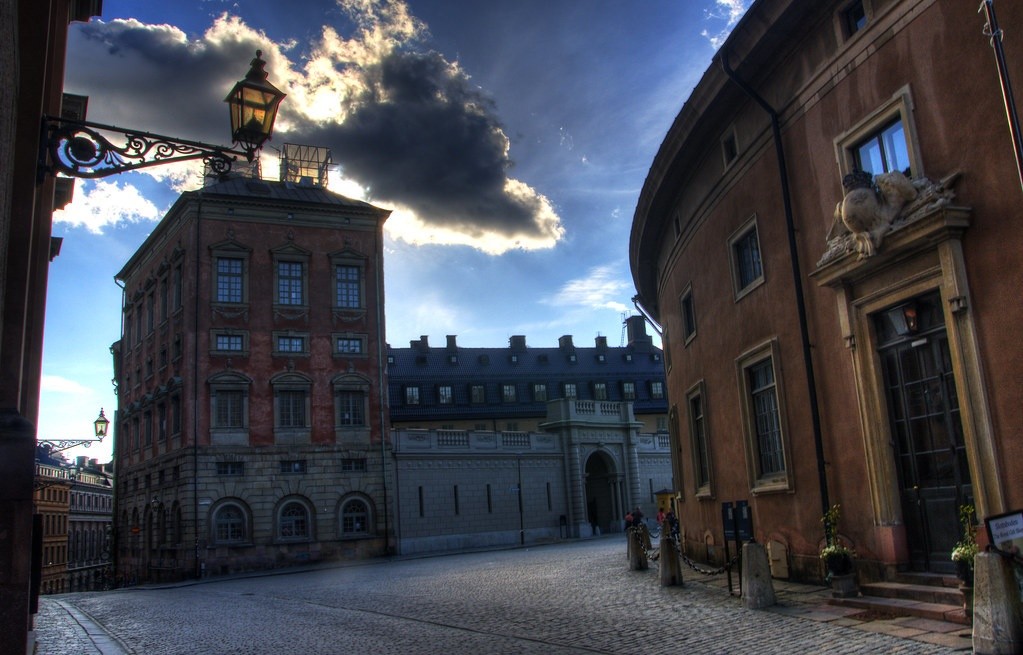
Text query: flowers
819 505 856 561
951 504 980 560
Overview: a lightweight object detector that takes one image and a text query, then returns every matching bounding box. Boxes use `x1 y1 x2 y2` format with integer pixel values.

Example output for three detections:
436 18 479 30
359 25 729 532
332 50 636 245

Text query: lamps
37 49 287 189
885 301 919 337
35 407 110 462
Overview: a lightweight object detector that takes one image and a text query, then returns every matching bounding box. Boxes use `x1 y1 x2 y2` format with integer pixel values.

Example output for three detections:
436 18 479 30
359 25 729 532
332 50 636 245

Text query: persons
657 506 680 542
624 507 643 532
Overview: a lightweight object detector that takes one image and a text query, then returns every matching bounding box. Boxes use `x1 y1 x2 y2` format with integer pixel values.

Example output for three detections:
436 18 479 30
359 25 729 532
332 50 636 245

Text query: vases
827 557 852 575
954 558 974 582
